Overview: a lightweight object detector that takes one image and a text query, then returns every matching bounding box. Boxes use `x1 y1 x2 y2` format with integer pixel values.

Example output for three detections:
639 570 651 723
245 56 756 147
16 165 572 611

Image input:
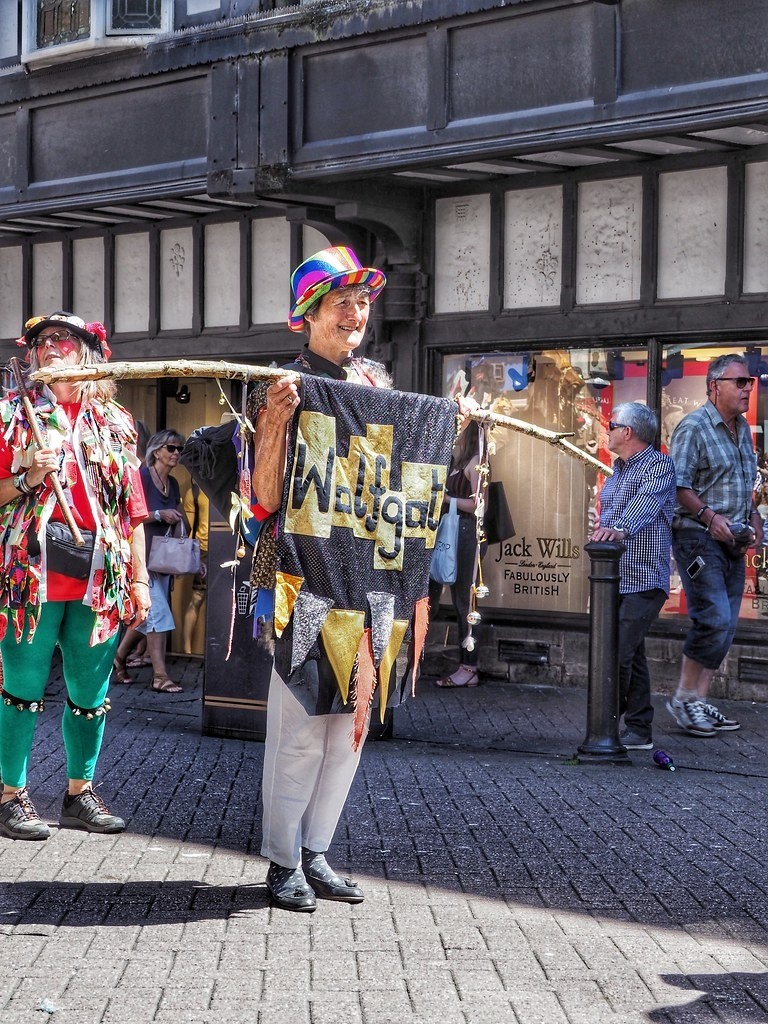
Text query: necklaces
153 463 168 492
725 422 735 436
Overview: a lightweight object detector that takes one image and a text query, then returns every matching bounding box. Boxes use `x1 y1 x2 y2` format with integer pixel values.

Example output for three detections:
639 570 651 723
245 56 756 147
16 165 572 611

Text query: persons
0 310 151 841
112 428 208 692
234 243 481 910
590 403 678 751
427 418 492 687
666 348 765 736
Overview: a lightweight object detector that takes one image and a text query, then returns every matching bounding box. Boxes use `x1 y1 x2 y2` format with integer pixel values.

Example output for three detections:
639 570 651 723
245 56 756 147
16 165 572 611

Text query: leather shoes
266 869 317 910
304 872 365 900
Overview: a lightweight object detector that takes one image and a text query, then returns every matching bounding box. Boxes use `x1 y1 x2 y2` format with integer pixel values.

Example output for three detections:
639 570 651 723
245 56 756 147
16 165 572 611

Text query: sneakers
0 786 51 839
59 782 125 831
666 695 717 736
677 704 740 730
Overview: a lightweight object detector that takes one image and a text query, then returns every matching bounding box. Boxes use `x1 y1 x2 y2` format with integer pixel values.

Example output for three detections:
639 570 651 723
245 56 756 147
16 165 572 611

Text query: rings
46 459 49 464
286 395 292 403
456 392 462 398
172 520 175 524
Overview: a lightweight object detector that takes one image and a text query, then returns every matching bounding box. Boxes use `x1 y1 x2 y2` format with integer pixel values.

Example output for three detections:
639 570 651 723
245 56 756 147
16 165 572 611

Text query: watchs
154 509 162 521
13 476 27 494
612 523 629 540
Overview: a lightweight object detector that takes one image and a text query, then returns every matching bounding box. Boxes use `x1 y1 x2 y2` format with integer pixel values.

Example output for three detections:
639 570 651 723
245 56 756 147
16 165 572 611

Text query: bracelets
749 508 760 521
131 579 149 586
19 471 34 494
697 506 709 519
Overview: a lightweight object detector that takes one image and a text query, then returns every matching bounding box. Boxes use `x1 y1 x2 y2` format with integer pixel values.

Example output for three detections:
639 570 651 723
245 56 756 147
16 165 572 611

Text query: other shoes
619 730 653 749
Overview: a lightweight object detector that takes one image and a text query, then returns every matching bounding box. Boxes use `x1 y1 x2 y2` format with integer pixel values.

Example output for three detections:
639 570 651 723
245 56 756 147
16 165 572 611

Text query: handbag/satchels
430 498 460 585
484 481 516 544
27 521 96 579
147 518 201 574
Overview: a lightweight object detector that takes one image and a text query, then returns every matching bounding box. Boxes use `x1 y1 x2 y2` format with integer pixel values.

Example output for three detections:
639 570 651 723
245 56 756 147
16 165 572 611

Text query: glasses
159 445 184 453
609 421 628 431
30 330 79 347
714 377 755 388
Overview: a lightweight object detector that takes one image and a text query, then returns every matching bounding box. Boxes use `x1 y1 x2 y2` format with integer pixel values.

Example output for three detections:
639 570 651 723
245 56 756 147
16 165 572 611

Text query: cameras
686 556 706 578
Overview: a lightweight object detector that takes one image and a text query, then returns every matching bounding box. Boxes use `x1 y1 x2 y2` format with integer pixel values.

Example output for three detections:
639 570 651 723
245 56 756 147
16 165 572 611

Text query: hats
288 246 386 332
15 310 112 363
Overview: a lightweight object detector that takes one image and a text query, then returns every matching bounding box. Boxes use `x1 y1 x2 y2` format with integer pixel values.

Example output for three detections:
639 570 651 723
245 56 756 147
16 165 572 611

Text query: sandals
150 673 183 693
435 665 479 688
114 652 131 683
